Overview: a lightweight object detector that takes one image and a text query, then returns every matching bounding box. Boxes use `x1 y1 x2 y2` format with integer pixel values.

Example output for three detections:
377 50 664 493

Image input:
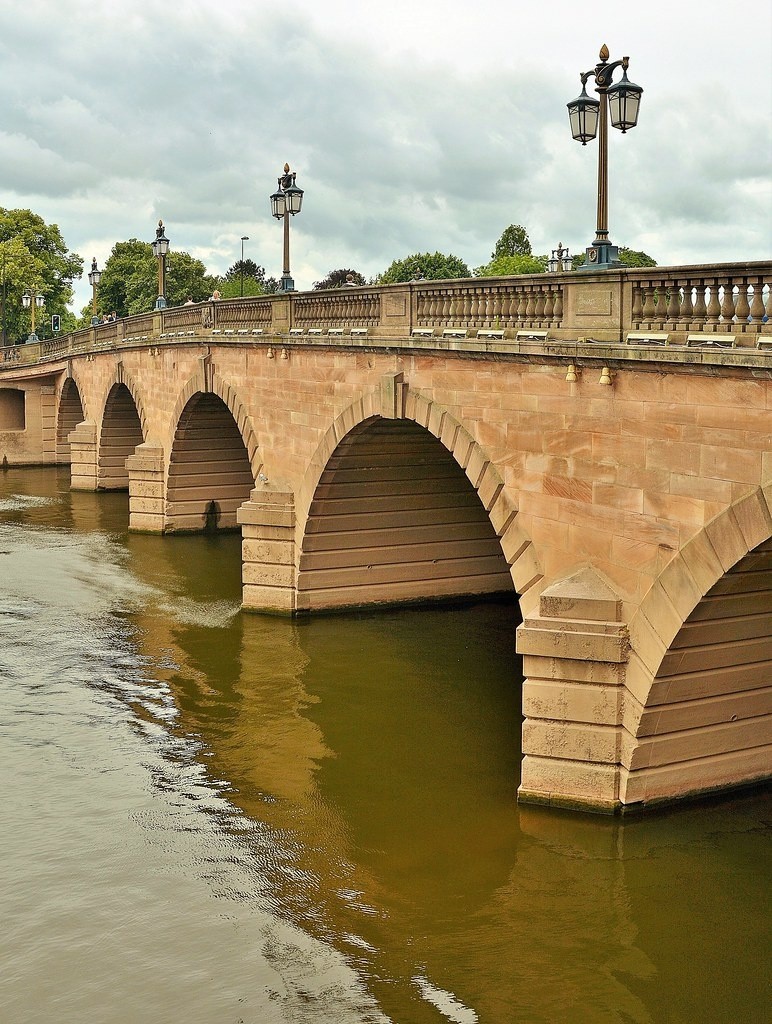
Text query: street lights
565 43 643 270
547 243 573 273
239 236 249 297
22 283 45 343
269 163 305 293
152 220 169 310
87 256 102 324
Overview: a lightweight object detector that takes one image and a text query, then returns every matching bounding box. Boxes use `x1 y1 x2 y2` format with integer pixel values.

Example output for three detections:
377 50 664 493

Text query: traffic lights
52 315 61 331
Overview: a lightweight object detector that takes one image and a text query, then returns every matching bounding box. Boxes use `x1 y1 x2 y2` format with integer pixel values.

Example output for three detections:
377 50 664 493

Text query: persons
108 311 119 322
100 315 109 325
207 290 222 300
341 274 357 287
183 296 195 306
